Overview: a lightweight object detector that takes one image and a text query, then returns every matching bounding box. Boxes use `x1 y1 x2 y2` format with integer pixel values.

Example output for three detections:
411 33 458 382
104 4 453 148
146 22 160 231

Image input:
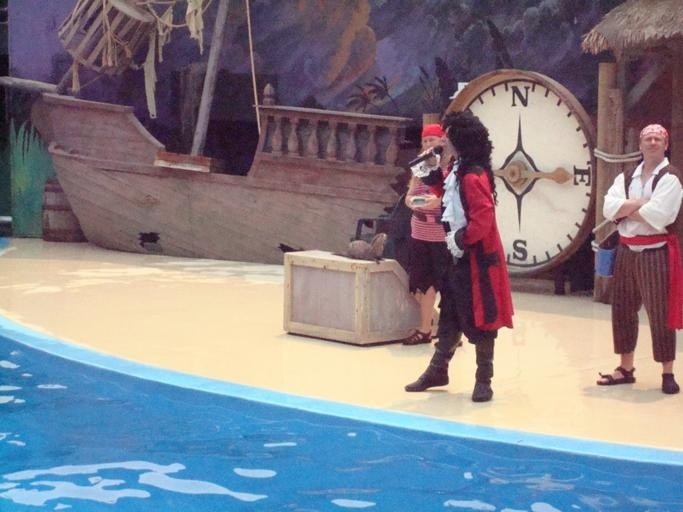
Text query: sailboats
30 0 414 263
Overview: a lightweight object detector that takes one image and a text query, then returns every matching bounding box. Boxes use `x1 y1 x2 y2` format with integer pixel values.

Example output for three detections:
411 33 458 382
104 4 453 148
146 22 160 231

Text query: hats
422 124 444 137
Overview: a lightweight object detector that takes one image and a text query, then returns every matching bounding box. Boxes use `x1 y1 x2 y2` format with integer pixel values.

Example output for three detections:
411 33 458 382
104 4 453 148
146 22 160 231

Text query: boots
472 339 495 400
405 331 461 391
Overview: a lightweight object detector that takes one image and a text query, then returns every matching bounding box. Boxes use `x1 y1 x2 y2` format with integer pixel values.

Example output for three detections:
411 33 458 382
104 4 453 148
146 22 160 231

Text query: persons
594 122 682 394
403 107 514 403
402 121 464 350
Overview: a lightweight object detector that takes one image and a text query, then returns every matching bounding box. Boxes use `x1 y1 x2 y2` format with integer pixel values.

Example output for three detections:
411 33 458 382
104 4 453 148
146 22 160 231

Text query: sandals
597 367 636 385
661 373 679 394
403 330 433 344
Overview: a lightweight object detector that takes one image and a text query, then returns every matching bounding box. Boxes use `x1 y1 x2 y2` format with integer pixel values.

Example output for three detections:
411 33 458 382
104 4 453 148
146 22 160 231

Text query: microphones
408 144 444 168
